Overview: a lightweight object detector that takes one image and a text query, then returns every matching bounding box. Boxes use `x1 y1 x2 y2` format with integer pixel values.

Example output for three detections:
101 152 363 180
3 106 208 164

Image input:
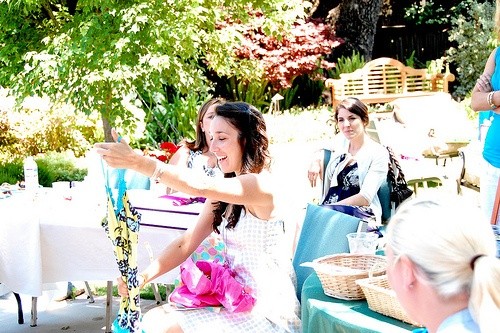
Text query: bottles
24 156 39 189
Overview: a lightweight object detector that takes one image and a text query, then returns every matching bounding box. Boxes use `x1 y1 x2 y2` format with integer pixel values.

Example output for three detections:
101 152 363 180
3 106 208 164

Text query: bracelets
487 91 497 110
151 161 164 181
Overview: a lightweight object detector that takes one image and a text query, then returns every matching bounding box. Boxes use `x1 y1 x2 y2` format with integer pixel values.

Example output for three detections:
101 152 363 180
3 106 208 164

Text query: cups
52 180 70 190
347 232 378 254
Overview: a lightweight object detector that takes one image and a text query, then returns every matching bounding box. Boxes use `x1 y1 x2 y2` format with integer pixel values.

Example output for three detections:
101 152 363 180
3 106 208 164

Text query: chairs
318 146 396 226
292 202 373 302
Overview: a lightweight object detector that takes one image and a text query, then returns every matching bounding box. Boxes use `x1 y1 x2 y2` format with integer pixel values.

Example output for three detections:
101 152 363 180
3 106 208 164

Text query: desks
296 246 425 333
0 181 222 333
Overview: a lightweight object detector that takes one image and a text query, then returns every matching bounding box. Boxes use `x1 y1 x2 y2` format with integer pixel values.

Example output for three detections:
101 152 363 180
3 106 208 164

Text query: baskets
445 141 469 152
355 275 424 328
313 252 389 301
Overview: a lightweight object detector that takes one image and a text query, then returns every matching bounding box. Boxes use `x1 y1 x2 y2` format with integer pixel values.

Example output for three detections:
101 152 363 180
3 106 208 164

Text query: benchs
324 56 458 128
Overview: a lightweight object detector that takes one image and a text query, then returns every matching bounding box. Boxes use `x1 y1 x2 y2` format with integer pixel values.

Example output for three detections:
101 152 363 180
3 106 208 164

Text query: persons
95 102 301 333
471 1 500 226
387 197 500 333
308 97 390 230
167 99 223 194
